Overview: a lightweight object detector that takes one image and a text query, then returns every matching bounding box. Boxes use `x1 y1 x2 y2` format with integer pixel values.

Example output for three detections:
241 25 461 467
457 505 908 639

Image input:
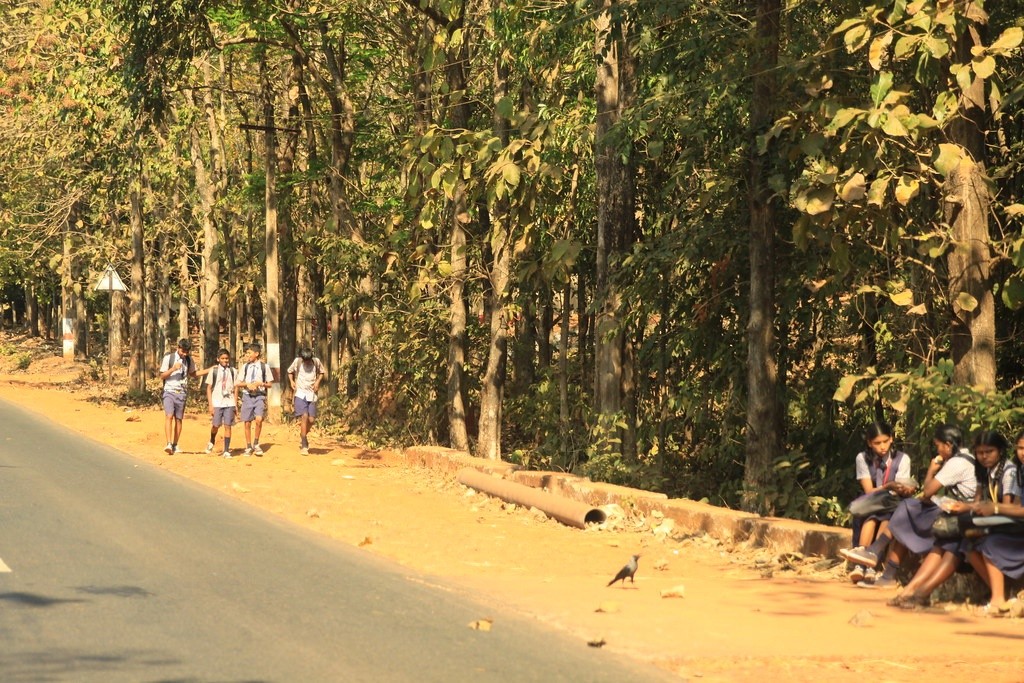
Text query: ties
222 370 226 394
251 365 255 382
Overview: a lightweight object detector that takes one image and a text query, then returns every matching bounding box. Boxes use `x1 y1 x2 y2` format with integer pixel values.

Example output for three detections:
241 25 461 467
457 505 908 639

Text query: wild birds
606 553 644 590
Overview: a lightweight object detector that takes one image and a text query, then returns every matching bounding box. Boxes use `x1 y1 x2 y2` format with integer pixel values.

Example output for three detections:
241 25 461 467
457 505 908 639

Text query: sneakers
206 442 213 454
252 444 263 456
244 447 252 457
165 444 172 454
223 452 232 458
173 445 182 453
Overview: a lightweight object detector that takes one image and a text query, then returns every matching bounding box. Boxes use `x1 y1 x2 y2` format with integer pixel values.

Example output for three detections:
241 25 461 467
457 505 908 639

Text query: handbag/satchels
932 514 975 540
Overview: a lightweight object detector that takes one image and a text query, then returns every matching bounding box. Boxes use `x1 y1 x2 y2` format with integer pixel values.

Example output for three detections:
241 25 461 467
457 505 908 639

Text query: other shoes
864 567 877 583
840 547 878 566
974 604 1010 618
888 595 918 608
858 575 899 590
901 598 932 612
847 563 862 582
302 447 309 456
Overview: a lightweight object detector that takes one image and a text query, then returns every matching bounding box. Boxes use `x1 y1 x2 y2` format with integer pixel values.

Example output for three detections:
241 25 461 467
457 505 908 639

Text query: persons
839 416 1023 608
204 349 240 458
234 343 275 456
158 338 217 455
286 347 325 456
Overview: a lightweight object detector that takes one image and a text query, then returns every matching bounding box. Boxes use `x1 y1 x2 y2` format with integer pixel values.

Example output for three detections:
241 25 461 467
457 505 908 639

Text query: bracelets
994 503 999 515
